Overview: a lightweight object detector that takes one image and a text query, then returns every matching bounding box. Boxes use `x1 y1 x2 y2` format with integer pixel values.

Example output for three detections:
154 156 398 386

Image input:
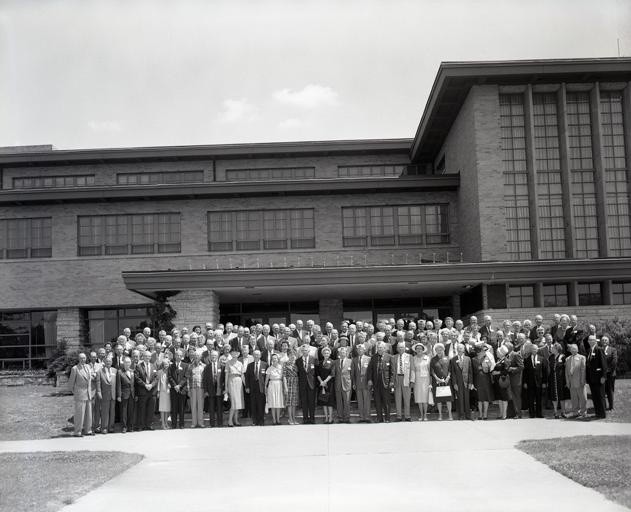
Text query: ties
77 334 609 382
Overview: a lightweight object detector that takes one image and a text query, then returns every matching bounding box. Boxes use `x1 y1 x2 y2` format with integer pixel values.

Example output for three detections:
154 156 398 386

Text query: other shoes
337 412 607 422
76 406 333 437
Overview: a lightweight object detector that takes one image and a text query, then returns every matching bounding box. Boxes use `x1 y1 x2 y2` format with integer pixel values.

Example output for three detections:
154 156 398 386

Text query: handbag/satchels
499 375 510 389
435 382 453 398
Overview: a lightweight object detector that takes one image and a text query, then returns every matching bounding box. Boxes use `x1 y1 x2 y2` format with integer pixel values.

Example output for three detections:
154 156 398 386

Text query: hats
337 335 349 344
498 345 509 356
411 343 427 353
433 343 445 352
